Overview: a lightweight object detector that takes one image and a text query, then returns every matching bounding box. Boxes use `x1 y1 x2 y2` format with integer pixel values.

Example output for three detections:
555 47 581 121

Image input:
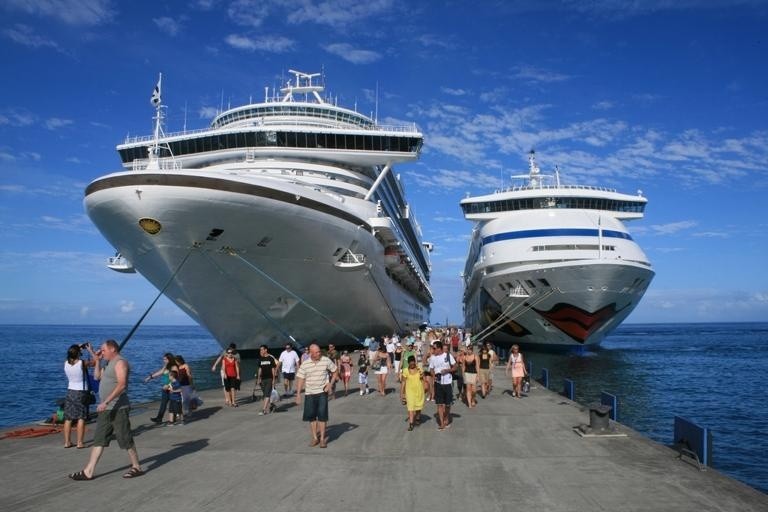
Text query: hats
396 343 402 347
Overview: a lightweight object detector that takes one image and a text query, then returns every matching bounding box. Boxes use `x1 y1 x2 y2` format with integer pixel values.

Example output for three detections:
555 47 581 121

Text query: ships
456 150 656 347
83 62 434 361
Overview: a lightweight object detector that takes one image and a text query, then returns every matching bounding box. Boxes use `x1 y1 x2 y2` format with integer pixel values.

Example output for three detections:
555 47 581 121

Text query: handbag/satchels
237 379 240 391
521 376 530 392
82 392 96 405
447 351 462 379
372 352 383 370
190 391 197 410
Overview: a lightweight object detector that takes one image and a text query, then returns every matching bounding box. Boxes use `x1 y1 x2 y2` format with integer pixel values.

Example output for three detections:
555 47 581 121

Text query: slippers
123 468 142 478
64 442 76 448
77 443 91 448
68 470 94 480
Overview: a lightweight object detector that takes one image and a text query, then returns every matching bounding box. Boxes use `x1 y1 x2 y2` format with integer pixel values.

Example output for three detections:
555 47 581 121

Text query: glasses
433 347 438 350
228 352 233 354
408 345 413 347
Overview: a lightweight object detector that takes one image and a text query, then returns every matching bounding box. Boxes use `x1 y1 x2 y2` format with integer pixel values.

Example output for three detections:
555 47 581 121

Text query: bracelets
150 375 154 379
104 400 109 404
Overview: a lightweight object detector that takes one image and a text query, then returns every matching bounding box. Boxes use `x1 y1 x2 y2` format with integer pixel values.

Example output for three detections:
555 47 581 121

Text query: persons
69 340 142 481
144 353 180 423
174 355 194 417
212 343 241 404
163 371 185 426
63 343 96 449
256 322 499 448
506 344 527 398
222 348 240 407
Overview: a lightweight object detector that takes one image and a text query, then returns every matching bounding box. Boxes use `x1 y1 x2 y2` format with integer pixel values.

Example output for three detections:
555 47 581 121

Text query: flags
150 80 161 107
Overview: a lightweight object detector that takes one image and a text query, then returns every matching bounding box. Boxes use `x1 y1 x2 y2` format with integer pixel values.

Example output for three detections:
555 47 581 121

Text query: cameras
77 341 89 352
436 372 441 381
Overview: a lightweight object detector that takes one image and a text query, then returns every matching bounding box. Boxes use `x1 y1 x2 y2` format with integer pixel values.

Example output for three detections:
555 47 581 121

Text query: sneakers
259 409 267 416
366 388 369 394
360 390 364 396
174 420 184 425
408 423 414 431
308 438 319 446
163 422 173 426
150 417 162 424
416 419 420 425
319 438 326 448
